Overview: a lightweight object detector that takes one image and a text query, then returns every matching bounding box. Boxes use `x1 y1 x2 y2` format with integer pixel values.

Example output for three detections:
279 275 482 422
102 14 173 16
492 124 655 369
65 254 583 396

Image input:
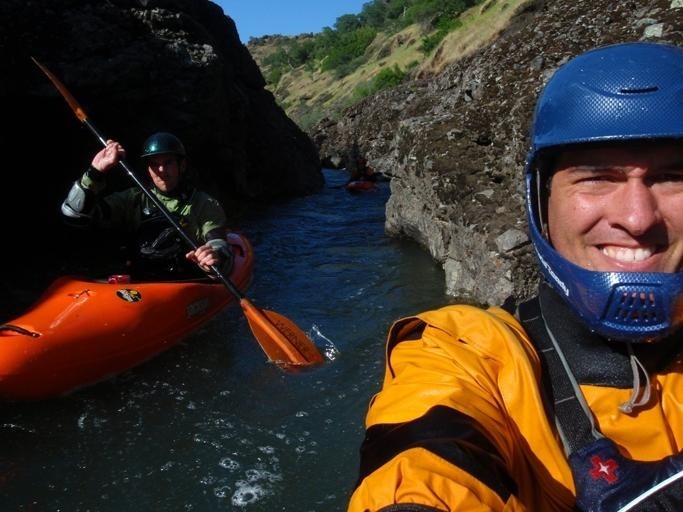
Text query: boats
1 228 258 406
344 180 375 192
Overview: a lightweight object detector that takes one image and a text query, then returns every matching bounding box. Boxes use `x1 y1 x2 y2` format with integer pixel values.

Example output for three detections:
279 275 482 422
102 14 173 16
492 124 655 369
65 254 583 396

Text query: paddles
30 56 325 372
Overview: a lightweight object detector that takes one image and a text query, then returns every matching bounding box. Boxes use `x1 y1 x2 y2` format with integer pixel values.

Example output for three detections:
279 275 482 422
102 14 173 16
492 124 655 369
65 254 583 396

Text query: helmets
141 133 185 165
524 42 682 344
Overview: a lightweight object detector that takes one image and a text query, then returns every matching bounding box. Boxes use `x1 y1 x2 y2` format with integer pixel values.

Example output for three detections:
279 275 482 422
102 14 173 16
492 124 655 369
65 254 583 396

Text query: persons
344 43 683 512
59 132 229 280
